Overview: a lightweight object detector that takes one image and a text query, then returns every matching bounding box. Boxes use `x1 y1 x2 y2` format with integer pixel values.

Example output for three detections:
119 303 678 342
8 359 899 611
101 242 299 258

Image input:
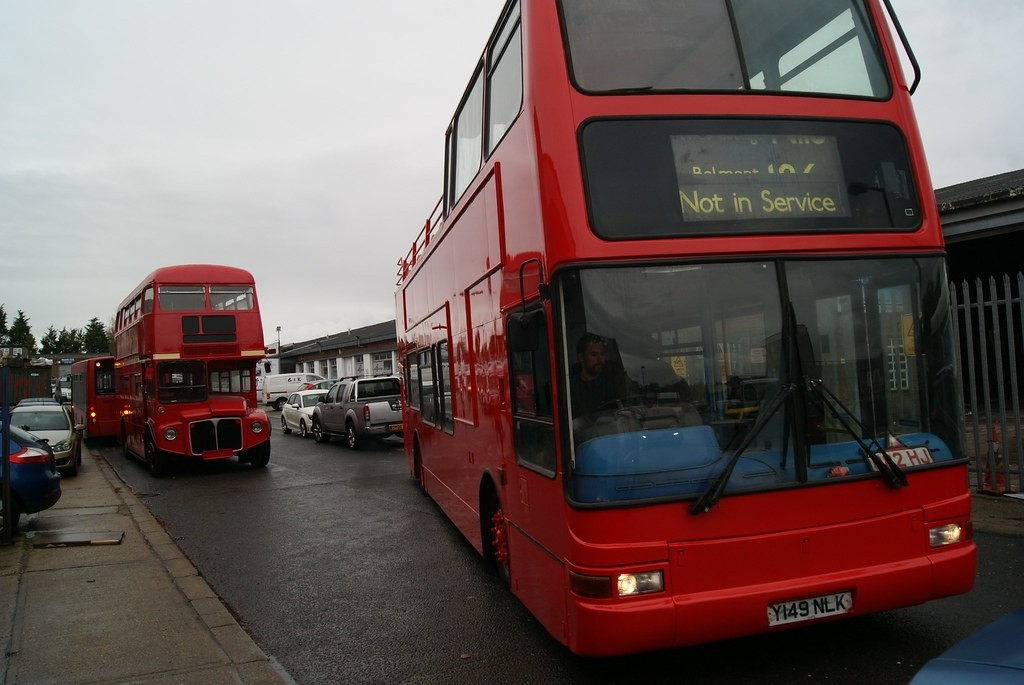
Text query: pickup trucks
310 372 407 451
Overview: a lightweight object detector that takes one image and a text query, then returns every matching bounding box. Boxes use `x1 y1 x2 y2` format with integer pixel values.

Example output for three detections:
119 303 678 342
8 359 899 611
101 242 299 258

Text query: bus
394 0 978 665
112 264 272 471
67 355 116 443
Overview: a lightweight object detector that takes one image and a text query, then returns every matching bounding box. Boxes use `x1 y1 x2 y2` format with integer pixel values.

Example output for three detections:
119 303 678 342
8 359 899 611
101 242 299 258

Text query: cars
51 384 56 393
280 390 330 439
296 378 339 393
0 419 62 541
8 396 87 476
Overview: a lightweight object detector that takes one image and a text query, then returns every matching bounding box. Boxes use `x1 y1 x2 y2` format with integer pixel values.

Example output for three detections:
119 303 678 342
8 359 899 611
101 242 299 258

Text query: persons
565 333 627 428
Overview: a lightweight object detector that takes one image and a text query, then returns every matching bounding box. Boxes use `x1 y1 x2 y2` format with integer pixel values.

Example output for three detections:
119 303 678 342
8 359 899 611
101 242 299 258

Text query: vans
55 377 71 404
262 373 331 411
